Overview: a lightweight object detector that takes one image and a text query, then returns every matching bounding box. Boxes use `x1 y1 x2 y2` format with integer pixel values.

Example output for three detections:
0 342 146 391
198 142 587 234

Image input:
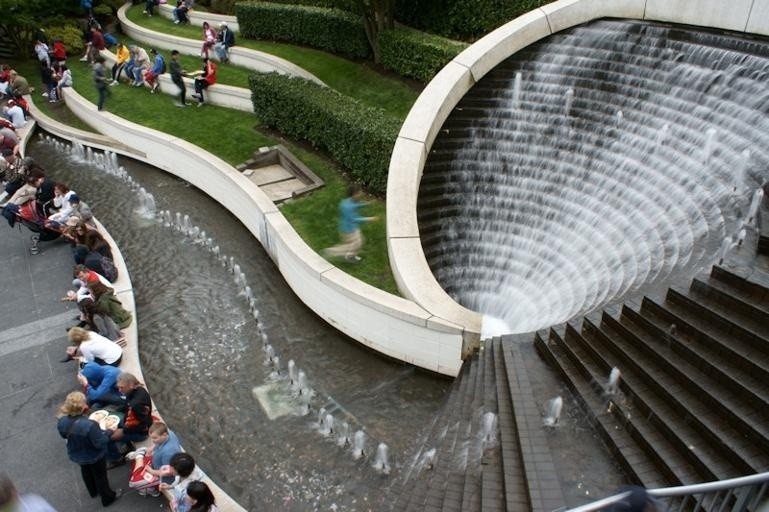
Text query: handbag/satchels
114 338 127 348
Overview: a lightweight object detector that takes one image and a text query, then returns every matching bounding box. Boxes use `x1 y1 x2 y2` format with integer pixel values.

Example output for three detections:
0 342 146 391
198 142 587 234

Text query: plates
100 414 119 430
88 410 108 422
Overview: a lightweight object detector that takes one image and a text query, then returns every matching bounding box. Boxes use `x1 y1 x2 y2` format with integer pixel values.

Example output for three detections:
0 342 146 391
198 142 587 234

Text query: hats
67 195 81 202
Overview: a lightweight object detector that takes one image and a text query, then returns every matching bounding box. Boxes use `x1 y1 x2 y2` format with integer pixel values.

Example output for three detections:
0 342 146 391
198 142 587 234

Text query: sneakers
113 488 122 499
138 488 160 497
109 80 119 86
80 58 88 62
191 93 201 98
41 92 49 96
125 447 146 460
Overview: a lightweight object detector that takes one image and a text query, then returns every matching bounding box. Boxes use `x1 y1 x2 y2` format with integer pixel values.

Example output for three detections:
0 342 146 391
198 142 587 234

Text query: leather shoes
59 356 74 362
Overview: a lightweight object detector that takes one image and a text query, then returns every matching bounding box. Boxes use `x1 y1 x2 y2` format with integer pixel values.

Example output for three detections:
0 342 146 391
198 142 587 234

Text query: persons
34 16 218 113
0 64 113 260
81 0 94 15
125 420 181 497
56 244 152 469
214 22 235 64
144 0 166 16
178 1 193 26
315 186 380 262
0 469 56 512
56 392 123 507
159 452 203 512
185 480 218 512
172 1 183 24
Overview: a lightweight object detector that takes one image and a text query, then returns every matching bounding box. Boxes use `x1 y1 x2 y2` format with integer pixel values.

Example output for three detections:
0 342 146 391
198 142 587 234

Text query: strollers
0 197 81 255
